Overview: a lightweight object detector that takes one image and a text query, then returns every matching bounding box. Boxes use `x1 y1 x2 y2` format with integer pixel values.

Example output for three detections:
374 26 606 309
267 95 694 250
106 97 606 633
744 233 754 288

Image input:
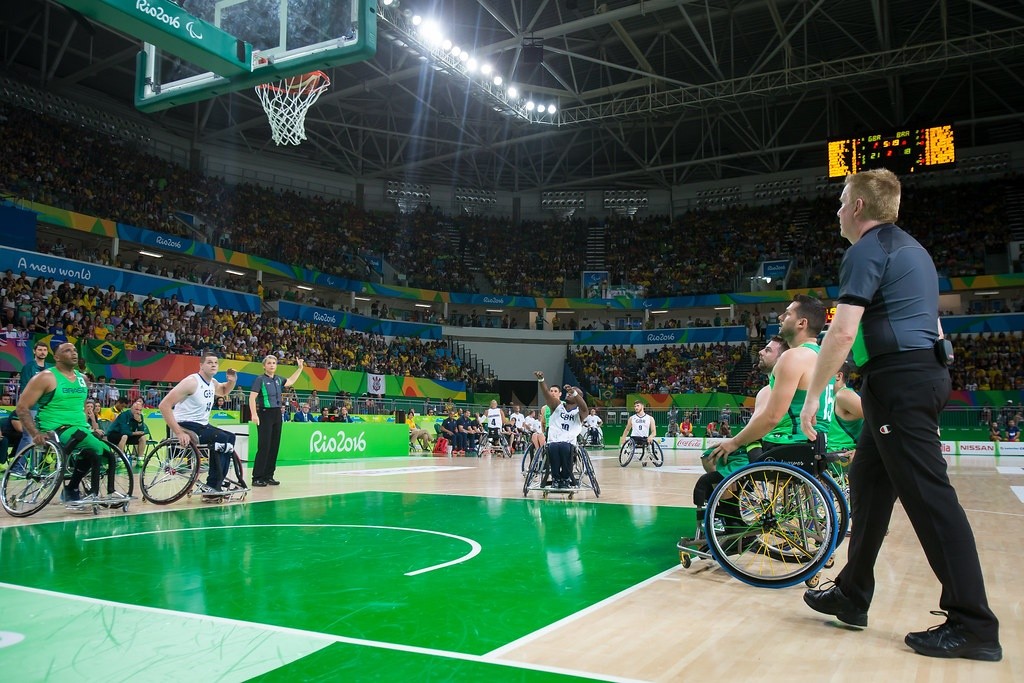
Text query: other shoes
423 448 430 452
559 479 569 487
431 439 437 443
202 495 229 502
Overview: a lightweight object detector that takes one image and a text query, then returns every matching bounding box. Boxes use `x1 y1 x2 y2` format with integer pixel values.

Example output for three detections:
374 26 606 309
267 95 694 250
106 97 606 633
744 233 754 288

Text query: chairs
411 425 535 453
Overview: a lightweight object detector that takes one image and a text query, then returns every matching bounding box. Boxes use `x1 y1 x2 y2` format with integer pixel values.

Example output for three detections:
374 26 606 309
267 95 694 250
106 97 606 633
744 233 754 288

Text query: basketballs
286 70 320 94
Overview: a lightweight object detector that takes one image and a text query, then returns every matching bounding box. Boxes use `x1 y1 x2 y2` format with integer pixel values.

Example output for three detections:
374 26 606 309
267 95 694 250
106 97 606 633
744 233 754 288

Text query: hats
303 404 310 408
1006 400 1013 404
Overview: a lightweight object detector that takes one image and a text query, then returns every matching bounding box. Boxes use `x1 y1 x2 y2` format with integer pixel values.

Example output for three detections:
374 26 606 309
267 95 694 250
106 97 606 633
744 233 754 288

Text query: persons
158 352 237 502
799 169 1003 662
534 370 589 488
16 343 129 503
703 293 867 558
10 342 51 478
249 355 305 486
0 102 1024 477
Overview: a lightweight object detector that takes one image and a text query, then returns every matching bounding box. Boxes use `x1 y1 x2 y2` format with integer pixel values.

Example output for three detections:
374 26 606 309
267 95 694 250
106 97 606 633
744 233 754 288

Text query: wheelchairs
138 424 252 505
583 427 605 449
618 436 664 468
0 429 135 519
476 427 512 459
676 431 857 590
521 431 601 501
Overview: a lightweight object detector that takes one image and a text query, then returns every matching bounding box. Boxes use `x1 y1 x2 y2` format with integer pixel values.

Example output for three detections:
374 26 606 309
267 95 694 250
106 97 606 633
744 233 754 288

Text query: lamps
383 0 556 113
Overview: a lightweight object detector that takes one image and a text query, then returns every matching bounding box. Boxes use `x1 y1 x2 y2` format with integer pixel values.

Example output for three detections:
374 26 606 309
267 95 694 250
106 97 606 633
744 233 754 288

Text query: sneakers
266 479 280 485
803 578 868 626
905 611 1003 662
699 535 759 558
117 461 127 469
700 519 725 531
135 461 148 469
59 486 80 503
10 471 27 478
741 507 752 517
252 481 267 486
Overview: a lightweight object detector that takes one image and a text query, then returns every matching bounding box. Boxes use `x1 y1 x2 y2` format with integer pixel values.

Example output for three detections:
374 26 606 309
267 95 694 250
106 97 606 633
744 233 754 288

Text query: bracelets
538 378 545 383
572 391 578 397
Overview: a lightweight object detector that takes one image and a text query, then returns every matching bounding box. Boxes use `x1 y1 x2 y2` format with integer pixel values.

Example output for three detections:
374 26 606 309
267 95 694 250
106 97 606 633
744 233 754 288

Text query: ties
305 414 307 421
